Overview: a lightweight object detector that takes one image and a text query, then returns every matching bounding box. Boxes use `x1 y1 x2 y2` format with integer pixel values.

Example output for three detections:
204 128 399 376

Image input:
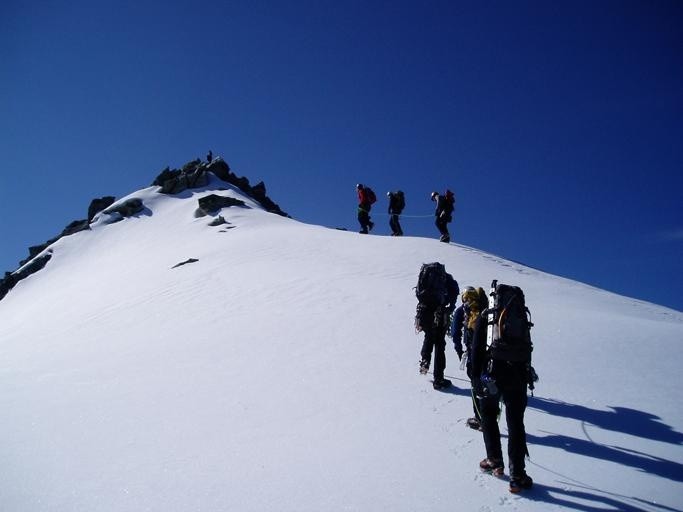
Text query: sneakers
391 231 403 236
467 417 484 433
433 378 451 391
480 455 503 470
440 233 450 242
369 222 374 231
359 230 368 234
509 471 532 493
420 359 429 371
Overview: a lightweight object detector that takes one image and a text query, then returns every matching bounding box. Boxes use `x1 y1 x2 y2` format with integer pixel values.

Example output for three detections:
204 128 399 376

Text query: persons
468 285 533 493
431 191 451 243
417 262 459 390
356 184 374 234
452 287 490 430
387 191 403 236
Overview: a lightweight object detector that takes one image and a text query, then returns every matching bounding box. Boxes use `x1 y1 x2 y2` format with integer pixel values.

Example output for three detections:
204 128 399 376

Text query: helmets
462 286 477 303
430 191 439 201
386 192 393 199
356 183 363 190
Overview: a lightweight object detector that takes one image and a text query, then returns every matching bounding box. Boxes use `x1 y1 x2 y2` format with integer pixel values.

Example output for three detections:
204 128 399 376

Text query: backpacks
483 283 534 378
364 187 376 204
394 190 405 210
415 262 447 305
439 188 455 222
